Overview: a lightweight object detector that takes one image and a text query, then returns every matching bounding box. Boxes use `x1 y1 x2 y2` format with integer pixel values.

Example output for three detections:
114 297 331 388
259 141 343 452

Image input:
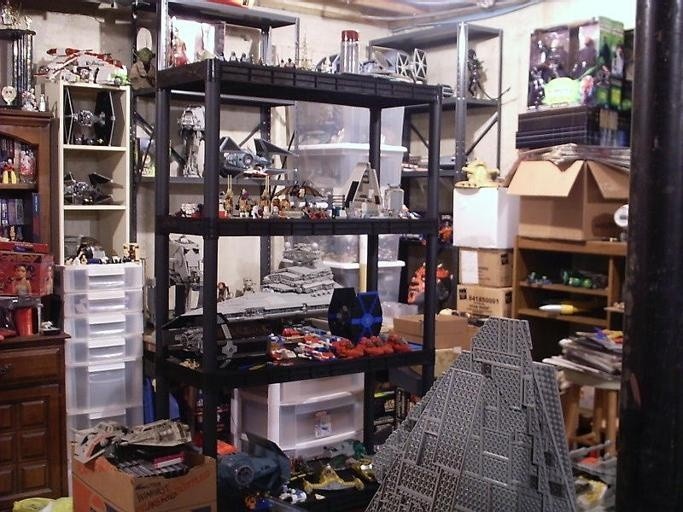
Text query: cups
340 30 359 74
13 306 34 336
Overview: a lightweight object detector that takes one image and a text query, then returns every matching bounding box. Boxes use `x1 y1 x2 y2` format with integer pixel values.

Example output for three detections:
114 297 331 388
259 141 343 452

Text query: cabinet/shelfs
152 62 444 511
31 73 141 435
509 232 631 434
0 0 636 512
1 107 72 512
125 2 303 437
367 20 503 319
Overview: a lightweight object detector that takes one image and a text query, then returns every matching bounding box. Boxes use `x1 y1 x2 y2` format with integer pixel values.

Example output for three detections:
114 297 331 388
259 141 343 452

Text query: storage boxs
503 160 630 248
452 185 519 351
393 310 469 385
